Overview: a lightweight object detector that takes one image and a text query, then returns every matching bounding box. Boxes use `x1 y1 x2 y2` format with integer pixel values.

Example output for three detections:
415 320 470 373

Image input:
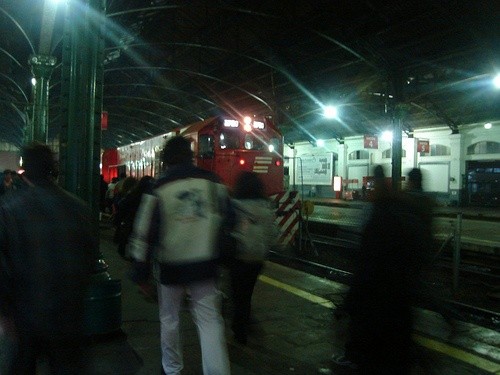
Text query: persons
222 169 277 344
0 141 100 375
326 164 445 368
103 172 163 231
0 165 25 198
126 135 256 375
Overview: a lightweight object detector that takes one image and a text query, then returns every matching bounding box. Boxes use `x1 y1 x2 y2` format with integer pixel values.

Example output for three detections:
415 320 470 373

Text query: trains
98 112 286 200
